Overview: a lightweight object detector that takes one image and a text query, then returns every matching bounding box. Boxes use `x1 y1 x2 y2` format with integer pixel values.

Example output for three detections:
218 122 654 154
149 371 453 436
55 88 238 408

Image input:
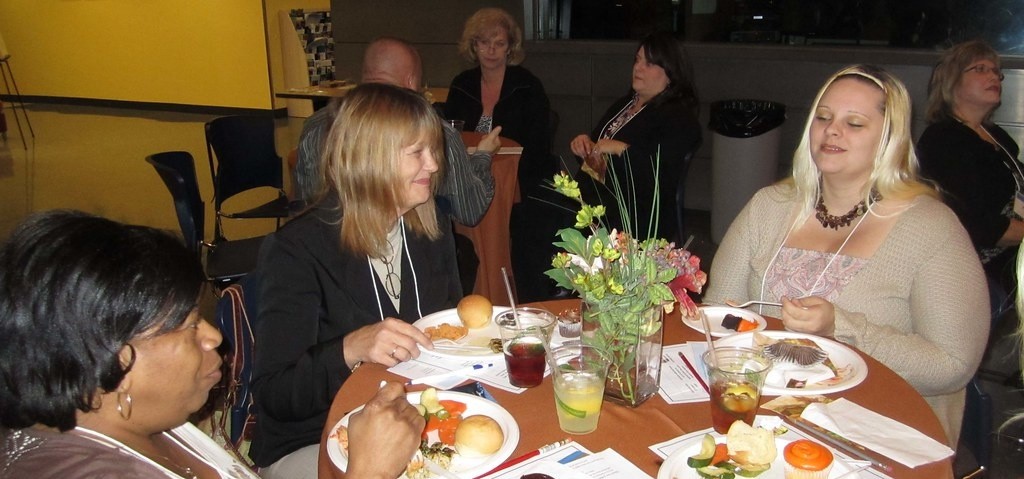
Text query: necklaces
815 176 883 231
141 435 198 479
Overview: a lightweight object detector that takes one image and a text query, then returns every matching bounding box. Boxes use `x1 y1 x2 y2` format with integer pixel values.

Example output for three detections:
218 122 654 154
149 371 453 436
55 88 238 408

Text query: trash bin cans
708 99 786 247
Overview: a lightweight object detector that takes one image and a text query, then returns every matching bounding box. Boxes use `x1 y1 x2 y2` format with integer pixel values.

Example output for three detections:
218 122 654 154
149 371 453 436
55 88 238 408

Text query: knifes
422 455 458 479
434 346 488 351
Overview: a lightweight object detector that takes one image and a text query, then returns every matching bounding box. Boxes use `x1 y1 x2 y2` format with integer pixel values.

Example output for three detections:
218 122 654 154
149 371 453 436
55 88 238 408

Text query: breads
727 420 777 465
457 295 493 329
454 415 504 455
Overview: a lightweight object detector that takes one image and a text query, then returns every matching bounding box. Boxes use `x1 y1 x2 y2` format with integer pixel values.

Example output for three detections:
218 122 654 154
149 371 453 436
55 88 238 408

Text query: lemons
560 387 601 418
719 382 758 412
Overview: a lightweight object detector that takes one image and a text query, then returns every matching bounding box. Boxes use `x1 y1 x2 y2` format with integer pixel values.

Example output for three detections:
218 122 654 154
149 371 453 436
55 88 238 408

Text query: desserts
558 309 581 337
721 314 758 331
783 439 834 479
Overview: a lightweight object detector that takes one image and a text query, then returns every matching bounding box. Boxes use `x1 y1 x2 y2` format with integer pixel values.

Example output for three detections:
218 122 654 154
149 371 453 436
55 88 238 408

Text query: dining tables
320 295 955 479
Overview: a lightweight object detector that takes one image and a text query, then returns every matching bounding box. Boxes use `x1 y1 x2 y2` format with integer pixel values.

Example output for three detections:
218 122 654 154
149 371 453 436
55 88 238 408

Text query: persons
979 238 1024 479
698 65 990 462
915 40 1024 313
431 7 547 151
0 208 427 479
247 82 464 467
541 28 702 299
295 37 502 296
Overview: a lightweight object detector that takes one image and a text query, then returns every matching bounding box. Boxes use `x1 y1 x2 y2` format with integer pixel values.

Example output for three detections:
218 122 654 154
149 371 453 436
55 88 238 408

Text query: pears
687 433 771 479
411 388 444 420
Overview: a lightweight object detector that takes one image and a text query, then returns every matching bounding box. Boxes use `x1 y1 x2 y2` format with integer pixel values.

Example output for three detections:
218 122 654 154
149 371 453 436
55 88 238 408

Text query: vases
580 301 664 408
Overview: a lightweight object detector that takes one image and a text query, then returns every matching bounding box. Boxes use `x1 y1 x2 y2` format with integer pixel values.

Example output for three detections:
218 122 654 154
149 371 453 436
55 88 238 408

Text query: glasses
959 63 1004 79
377 239 403 299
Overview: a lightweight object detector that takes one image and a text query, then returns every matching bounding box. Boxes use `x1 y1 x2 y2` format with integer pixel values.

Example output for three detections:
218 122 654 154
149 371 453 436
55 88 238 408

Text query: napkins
799 397 955 468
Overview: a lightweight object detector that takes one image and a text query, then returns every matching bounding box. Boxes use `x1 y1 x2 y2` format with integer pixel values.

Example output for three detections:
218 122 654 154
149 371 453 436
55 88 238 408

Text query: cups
702 346 772 435
546 345 614 435
495 308 557 388
319 82 330 93
446 120 465 137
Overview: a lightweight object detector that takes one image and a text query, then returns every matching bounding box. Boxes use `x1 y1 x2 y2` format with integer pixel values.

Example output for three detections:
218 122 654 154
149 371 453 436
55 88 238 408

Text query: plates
657 435 861 479
411 306 516 359
713 331 868 395
682 306 767 337
327 391 520 479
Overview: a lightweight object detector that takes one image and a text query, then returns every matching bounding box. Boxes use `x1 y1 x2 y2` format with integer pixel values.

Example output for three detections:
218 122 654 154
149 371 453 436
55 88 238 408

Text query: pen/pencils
678 353 712 393
472 437 572 479
784 413 893 474
405 363 493 385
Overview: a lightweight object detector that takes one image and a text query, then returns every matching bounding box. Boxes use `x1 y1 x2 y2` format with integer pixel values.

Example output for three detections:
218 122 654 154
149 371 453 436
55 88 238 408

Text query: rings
594 150 597 153
392 345 398 355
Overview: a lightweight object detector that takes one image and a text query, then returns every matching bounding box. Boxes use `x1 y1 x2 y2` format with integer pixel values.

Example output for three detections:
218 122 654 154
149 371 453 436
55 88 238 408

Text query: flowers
527 140 707 325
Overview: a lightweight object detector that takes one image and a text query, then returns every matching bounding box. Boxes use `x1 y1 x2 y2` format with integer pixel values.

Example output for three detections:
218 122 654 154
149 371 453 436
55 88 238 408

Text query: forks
727 301 783 307
381 380 424 475
432 335 472 346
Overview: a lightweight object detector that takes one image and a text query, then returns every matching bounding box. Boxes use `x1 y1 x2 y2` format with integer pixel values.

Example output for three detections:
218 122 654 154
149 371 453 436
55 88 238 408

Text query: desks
276 85 453 113
288 130 523 308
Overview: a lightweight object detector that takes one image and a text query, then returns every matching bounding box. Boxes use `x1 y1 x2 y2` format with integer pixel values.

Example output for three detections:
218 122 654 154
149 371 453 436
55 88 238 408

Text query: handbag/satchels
197 284 264 479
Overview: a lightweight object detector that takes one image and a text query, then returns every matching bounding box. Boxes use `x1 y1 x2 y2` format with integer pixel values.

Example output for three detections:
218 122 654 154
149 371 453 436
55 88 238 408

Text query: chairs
144 150 268 299
203 113 316 238
951 276 1011 479
661 139 705 253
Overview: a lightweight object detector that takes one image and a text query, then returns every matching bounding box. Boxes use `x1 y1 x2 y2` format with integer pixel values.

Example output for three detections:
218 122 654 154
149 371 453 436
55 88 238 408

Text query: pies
337 425 454 479
489 338 503 353
